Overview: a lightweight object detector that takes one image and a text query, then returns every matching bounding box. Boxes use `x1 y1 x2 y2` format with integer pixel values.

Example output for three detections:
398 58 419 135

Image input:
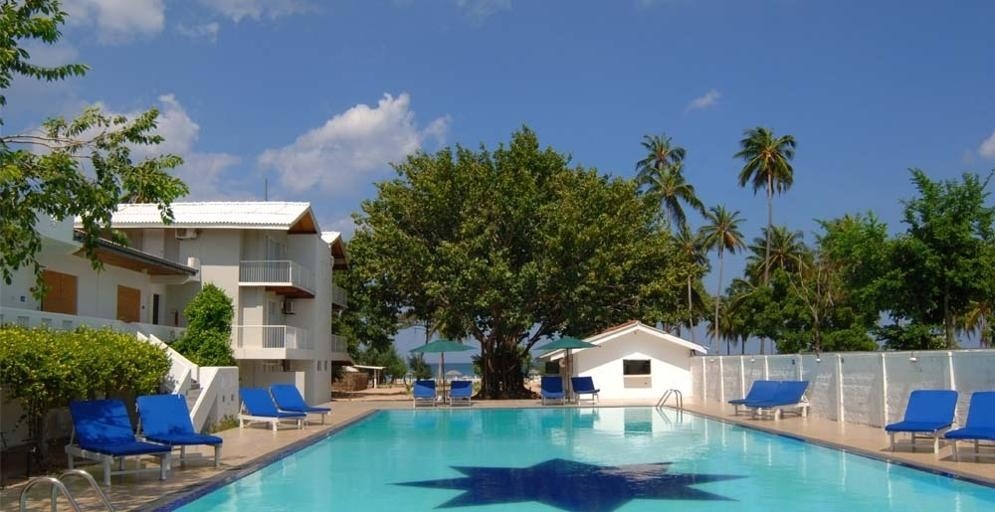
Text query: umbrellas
533 333 602 403
408 337 479 402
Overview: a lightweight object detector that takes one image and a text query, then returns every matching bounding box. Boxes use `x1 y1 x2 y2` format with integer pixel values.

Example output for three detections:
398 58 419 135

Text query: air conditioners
282 301 296 315
174 228 197 239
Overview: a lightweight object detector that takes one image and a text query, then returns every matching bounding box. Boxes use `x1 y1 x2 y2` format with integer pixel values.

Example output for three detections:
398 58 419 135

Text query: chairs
886 389 958 455
62 400 171 487
238 383 331 433
728 380 809 423
944 390 995 461
413 381 436 408
134 394 223 471
450 381 473 407
540 376 601 406
